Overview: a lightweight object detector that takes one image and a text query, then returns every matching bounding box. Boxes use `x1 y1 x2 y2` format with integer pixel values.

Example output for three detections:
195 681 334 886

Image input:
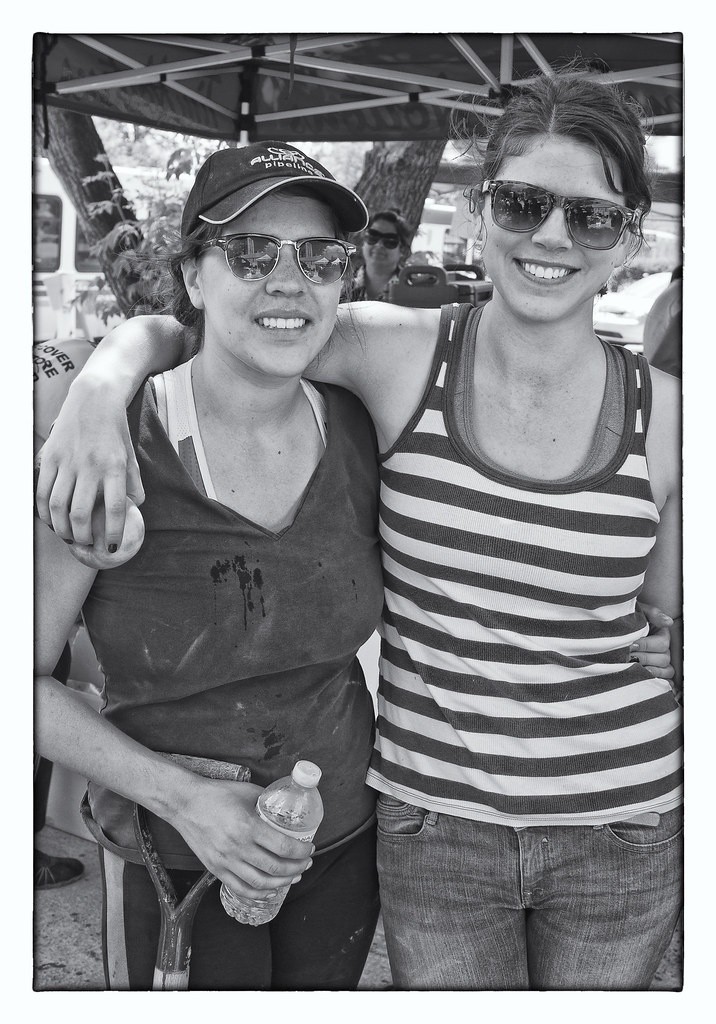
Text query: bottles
220 760 324 925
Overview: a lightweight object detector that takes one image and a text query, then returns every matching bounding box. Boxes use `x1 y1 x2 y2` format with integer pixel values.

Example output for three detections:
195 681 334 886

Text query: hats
180 139 369 254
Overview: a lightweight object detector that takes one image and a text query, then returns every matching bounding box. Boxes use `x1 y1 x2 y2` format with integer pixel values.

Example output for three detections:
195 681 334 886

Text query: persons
34 78 680 988
340 206 410 304
33 143 676 991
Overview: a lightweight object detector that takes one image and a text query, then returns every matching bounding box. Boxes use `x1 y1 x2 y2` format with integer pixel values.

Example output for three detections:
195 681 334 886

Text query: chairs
440 265 488 281
386 267 474 309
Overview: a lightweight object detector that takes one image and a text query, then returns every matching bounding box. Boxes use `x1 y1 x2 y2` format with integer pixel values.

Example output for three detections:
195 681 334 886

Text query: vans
35 154 125 340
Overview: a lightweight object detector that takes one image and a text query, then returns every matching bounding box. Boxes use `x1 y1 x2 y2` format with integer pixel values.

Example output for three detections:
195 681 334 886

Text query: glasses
364 228 401 249
481 179 645 250
195 233 357 286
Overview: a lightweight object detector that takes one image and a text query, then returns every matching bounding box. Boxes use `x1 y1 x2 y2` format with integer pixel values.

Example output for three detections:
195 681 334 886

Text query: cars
594 270 683 352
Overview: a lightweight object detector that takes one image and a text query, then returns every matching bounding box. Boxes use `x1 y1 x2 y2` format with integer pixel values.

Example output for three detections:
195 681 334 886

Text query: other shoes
33 848 85 889
